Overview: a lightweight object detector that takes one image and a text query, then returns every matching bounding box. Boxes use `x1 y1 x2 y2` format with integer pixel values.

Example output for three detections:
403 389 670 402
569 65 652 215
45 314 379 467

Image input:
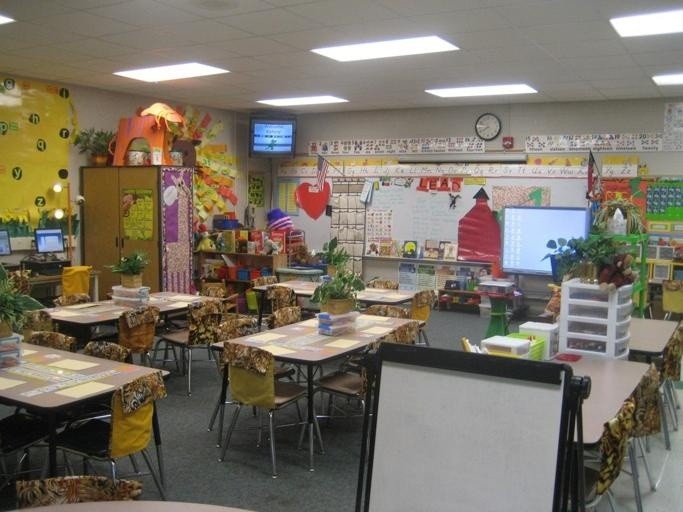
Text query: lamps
53 182 87 259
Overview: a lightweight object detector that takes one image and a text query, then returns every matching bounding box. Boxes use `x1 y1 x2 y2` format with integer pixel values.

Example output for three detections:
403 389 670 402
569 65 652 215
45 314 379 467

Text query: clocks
475 112 501 141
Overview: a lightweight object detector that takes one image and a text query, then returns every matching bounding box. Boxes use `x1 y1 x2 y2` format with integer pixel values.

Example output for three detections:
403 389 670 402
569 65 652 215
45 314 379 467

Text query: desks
8 501 255 512
0 342 171 479
546 351 652 448
567 318 683 512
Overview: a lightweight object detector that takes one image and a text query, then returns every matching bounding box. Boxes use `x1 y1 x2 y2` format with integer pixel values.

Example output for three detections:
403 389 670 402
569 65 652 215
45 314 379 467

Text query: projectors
479 281 515 295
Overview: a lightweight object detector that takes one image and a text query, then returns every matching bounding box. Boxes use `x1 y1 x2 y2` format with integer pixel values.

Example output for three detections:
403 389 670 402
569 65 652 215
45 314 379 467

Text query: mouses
32 272 39 276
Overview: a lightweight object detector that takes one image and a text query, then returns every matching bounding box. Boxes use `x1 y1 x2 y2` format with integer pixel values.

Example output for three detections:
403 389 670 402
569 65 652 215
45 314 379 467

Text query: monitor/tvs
0 230 12 255
249 114 296 159
34 228 65 253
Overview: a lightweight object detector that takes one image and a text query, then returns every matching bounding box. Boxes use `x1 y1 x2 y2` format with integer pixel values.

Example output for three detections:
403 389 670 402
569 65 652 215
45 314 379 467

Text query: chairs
295 334 397 455
254 276 276 309
331 323 419 414
275 307 325 412
339 308 408 402
226 342 325 479
635 279 653 318
217 320 307 449
363 279 399 312
54 368 168 501
8 266 222 375
14 476 144 508
265 287 308 321
662 280 683 320
404 289 436 347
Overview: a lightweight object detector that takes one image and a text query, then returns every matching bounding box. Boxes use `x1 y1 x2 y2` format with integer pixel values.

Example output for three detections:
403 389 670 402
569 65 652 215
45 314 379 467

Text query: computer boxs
0 261 20 272
20 259 71 275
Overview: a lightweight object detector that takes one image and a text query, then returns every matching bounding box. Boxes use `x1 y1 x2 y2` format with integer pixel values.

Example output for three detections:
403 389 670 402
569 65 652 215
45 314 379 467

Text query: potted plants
316 238 352 277
111 249 150 289
1 264 44 339
72 129 115 166
594 189 646 235
309 271 363 313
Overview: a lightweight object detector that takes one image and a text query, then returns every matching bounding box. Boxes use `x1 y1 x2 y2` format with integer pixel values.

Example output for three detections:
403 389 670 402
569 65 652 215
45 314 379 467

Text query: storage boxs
276 268 323 282
477 280 515 320
520 321 559 359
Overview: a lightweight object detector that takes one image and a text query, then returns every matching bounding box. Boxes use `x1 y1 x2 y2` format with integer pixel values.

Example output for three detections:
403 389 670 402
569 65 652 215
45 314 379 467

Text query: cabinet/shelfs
197 250 287 317
481 335 530 360
559 278 634 364
588 231 648 318
438 290 523 317
271 229 308 268
81 165 161 301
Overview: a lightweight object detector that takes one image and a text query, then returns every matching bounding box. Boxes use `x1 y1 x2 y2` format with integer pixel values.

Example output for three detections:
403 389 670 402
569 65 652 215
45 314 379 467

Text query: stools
1 413 53 488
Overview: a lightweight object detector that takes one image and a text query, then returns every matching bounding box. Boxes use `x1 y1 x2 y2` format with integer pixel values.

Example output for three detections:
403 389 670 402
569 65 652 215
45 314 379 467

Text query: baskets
493 333 544 360
